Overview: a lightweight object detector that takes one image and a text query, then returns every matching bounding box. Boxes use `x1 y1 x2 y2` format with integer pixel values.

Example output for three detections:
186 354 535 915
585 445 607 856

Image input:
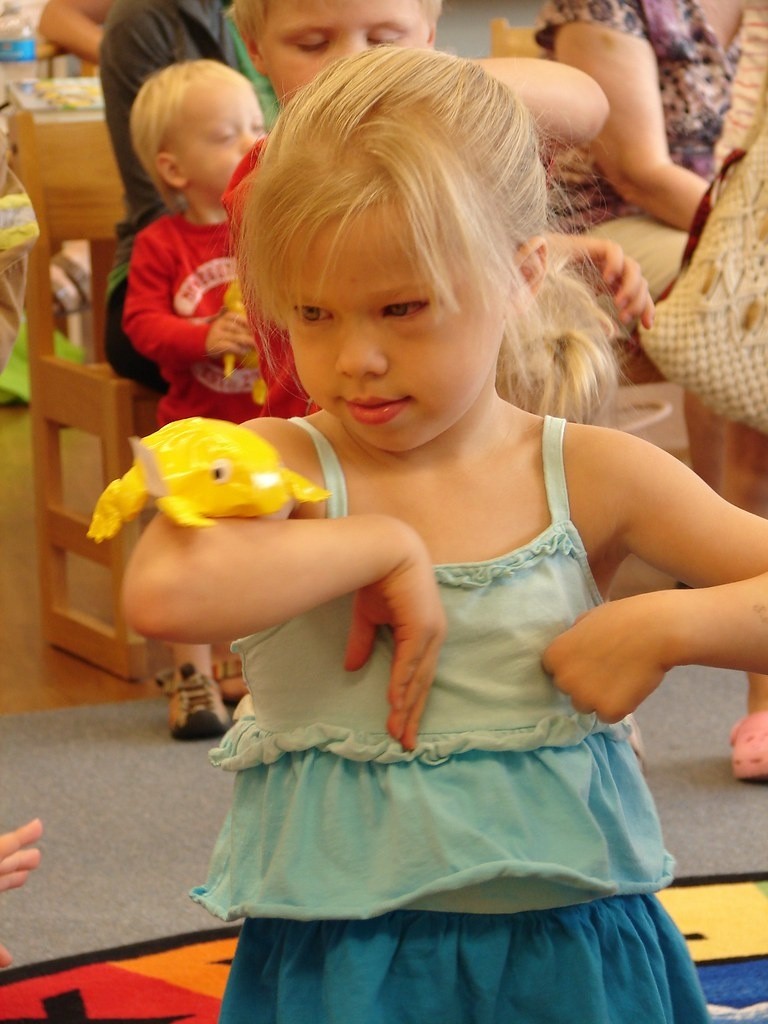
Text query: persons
119 49 768 1024
707 1 768 775
0 816 45 968
0 0 655 743
531 0 726 494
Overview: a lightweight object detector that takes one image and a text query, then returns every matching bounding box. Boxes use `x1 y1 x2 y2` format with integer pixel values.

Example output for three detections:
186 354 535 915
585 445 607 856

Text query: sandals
729 710 768 780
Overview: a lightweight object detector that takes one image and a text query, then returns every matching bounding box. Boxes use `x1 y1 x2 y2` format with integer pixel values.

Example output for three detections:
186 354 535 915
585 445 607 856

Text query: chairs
10 113 172 681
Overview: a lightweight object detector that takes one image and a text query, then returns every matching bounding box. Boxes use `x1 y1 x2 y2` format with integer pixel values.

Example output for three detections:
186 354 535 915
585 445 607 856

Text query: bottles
0 2 38 92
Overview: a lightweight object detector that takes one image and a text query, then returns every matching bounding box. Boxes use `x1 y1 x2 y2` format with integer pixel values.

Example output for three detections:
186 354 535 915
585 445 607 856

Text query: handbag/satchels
635 78 768 433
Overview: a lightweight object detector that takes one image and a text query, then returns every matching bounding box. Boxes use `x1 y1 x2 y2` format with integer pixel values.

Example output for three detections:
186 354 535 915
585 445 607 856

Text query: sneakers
163 664 232 739
